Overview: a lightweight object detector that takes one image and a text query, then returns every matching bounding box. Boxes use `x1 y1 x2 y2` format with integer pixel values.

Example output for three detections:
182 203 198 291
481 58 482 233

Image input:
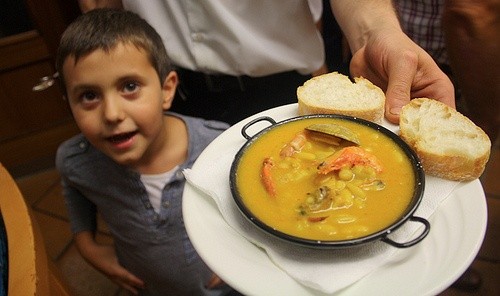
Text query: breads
399 97 491 184
297 71 386 127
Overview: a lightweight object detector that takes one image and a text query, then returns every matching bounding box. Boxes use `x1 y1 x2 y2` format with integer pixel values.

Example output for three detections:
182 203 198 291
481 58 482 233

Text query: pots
229 111 431 251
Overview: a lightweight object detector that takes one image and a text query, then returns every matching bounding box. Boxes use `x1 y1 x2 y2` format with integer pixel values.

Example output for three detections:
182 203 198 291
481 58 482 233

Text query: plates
183 99 488 296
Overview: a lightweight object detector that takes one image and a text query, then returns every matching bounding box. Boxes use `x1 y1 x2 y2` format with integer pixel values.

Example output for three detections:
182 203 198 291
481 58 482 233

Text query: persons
60 10 242 295
81 1 456 128
392 1 500 289
